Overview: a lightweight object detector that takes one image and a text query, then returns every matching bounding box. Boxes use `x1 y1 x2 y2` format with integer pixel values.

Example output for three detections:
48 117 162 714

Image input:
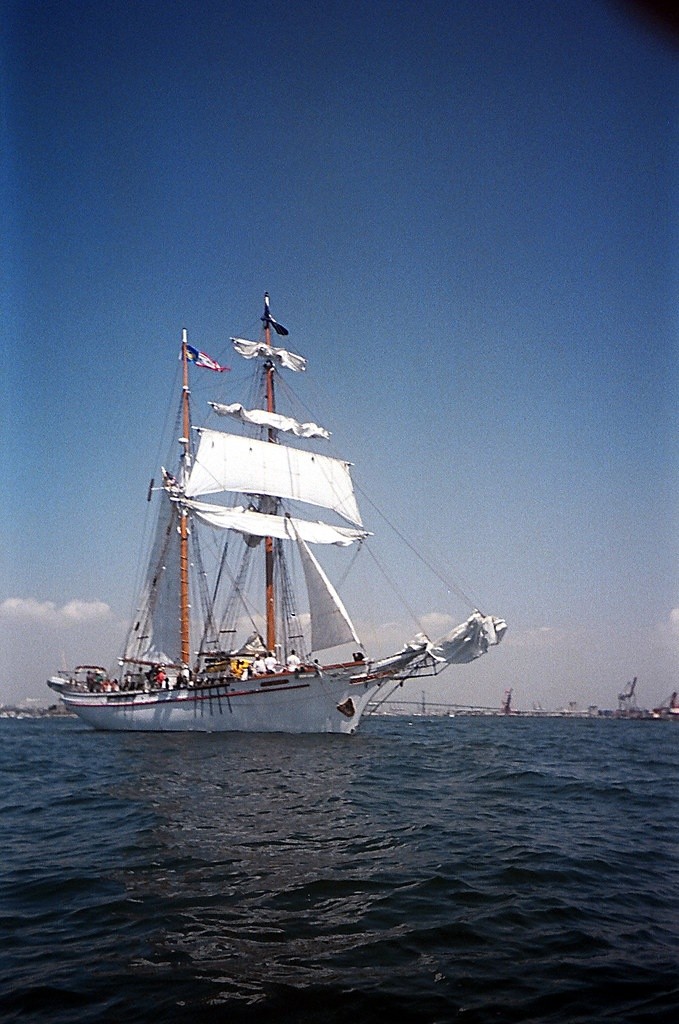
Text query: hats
158 668 162 671
255 654 259 657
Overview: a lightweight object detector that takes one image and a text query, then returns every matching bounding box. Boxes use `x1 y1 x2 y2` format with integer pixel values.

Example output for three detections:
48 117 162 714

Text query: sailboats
45 289 511 733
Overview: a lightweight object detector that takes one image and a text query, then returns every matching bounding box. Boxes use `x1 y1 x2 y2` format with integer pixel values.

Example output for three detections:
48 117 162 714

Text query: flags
178 343 231 372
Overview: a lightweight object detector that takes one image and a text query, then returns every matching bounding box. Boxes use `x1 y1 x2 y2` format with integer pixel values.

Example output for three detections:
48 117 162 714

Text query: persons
86 649 321 693
